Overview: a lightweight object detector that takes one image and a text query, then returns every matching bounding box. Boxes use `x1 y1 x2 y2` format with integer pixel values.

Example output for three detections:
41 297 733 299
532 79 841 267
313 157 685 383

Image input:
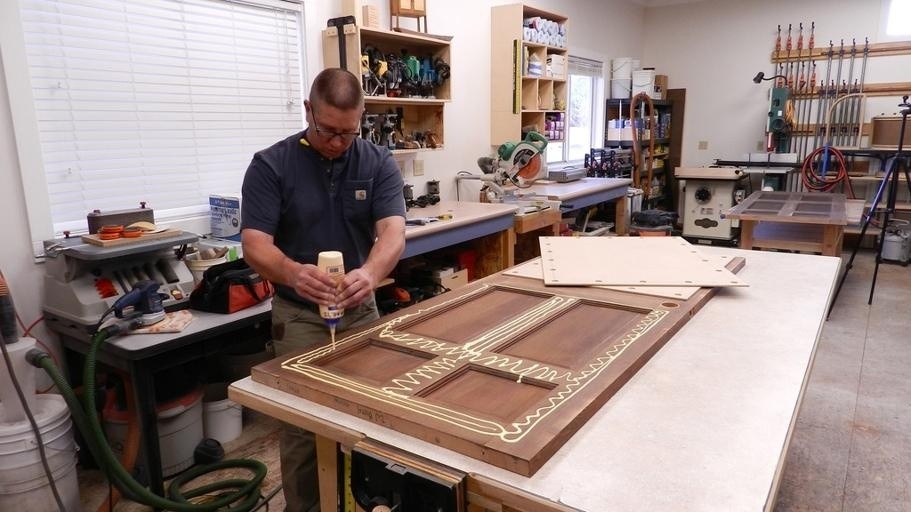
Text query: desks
722 191 847 256
228 245 841 512
53 295 275 510
397 203 518 277
479 177 634 245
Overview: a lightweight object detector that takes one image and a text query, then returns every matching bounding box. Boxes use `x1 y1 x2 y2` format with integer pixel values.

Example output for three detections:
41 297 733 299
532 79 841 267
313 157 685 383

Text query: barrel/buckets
184 252 231 287
99 376 205 479
201 379 243 443
610 58 655 100
220 348 273 378
0 392 82 512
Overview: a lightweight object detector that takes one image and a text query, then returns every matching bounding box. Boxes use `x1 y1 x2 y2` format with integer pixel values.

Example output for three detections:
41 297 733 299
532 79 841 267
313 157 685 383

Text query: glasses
310 103 360 144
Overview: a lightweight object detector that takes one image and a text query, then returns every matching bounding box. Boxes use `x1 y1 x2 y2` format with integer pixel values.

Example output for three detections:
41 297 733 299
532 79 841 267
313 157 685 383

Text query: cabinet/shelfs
818 147 911 236
605 99 673 210
320 26 452 156
492 3 570 148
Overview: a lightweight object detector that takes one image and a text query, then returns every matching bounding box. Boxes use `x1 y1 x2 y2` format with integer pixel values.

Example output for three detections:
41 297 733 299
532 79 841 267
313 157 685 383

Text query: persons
240 69 407 511
